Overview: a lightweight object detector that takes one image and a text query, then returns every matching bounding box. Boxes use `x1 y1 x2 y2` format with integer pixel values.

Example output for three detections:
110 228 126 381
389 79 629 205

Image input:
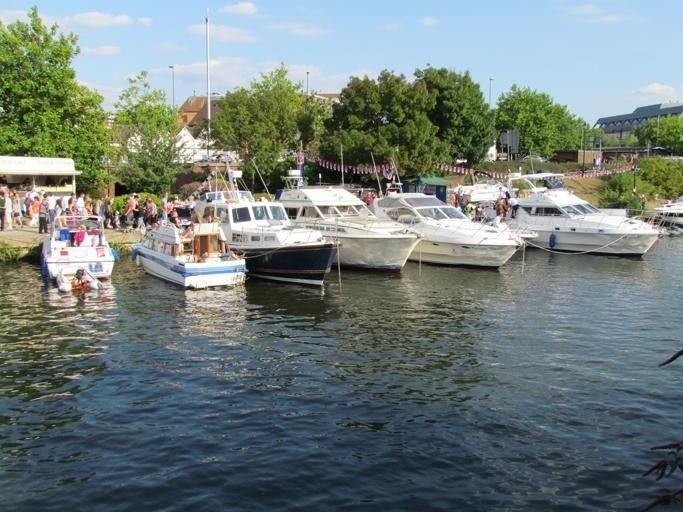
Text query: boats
504 188 683 258
370 193 522 271
272 184 423 273
39 215 117 288
191 163 340 288
132 223 244 291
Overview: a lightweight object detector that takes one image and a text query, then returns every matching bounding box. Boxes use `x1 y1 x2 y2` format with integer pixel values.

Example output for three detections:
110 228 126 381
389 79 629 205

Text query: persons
161 194 194 226
493 186 519 222
463 201 487 222
73 224 86 246
450 186 469 211
0 175 158 233
363 191 375 205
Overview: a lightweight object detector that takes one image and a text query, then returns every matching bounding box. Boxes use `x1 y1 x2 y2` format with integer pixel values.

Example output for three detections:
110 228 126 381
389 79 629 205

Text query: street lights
167 65 179 111
203 14 212 145
